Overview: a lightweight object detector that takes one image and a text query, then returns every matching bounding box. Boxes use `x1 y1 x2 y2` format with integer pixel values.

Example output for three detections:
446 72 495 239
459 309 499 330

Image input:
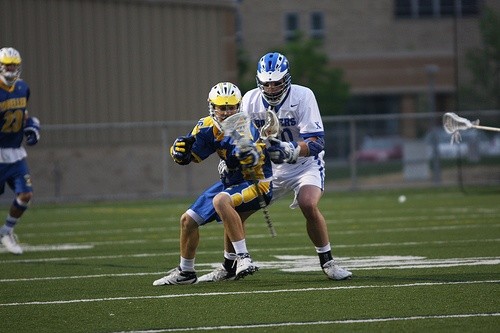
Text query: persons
198 51 353 281
0 47 41 255
152 82 279 286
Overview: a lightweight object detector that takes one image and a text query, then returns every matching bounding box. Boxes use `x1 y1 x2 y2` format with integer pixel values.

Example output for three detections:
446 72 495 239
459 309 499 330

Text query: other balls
398 195 406 203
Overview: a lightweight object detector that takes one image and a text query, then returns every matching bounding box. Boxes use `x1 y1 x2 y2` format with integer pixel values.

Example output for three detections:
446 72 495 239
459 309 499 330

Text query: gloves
23 117 41 145
230 148 258 168
170 136 196 165
265 137 301 164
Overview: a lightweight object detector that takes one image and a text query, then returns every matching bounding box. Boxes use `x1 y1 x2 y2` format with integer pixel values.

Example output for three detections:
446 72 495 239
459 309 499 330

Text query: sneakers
0 232 22 254
153 266 198 286
229 253 258 279
198 257 237 282
317 250 353 280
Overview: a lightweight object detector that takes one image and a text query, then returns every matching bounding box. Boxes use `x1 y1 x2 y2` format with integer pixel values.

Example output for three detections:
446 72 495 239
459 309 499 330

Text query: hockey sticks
442 111 500 134
221 111 277 237
260 112 280 140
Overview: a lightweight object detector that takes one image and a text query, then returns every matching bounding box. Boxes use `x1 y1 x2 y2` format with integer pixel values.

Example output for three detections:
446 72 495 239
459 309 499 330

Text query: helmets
256 52 291 105
0 47 21 86
207 82 242 132
